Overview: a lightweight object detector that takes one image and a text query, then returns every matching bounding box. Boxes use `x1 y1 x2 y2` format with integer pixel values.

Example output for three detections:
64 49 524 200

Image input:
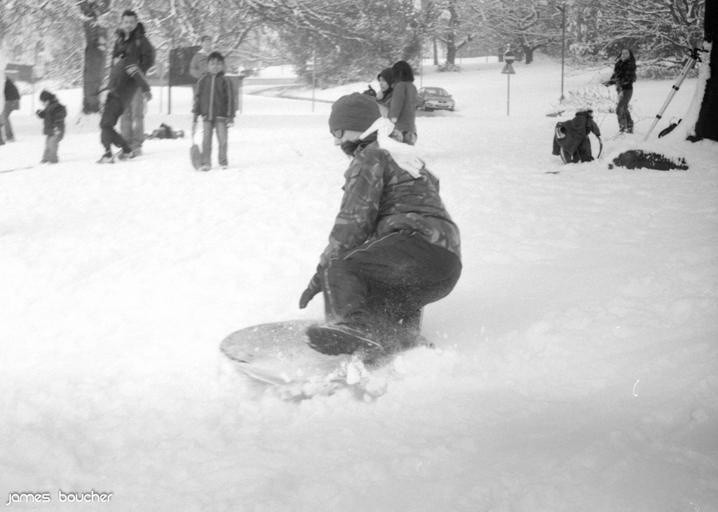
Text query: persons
296 88 463 370
0 73 24 146
601 47 641 135
33 88 68 164
375 67 395 110
549 107 605 164
97 51 154 166
186 34 217 88
386 59 421 146
107 8 158 157
192 51 238 172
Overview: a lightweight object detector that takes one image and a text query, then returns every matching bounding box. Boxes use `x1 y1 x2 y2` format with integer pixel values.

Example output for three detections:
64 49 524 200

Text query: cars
417 86 455 112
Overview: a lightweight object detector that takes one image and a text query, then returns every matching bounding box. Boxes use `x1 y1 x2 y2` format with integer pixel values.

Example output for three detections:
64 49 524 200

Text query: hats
328 92 380 131
40 92 52 102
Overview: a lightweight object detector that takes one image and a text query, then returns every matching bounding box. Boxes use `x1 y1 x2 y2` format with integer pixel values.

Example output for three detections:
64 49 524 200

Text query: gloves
300 263 325 309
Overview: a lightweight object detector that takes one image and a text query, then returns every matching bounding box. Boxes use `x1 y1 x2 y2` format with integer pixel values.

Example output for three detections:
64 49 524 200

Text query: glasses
332 129 343 138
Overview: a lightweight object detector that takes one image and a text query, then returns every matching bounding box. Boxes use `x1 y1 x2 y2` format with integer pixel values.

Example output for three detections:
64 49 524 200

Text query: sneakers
115 149 133 159
305 319 385 365
96 156 113 164
